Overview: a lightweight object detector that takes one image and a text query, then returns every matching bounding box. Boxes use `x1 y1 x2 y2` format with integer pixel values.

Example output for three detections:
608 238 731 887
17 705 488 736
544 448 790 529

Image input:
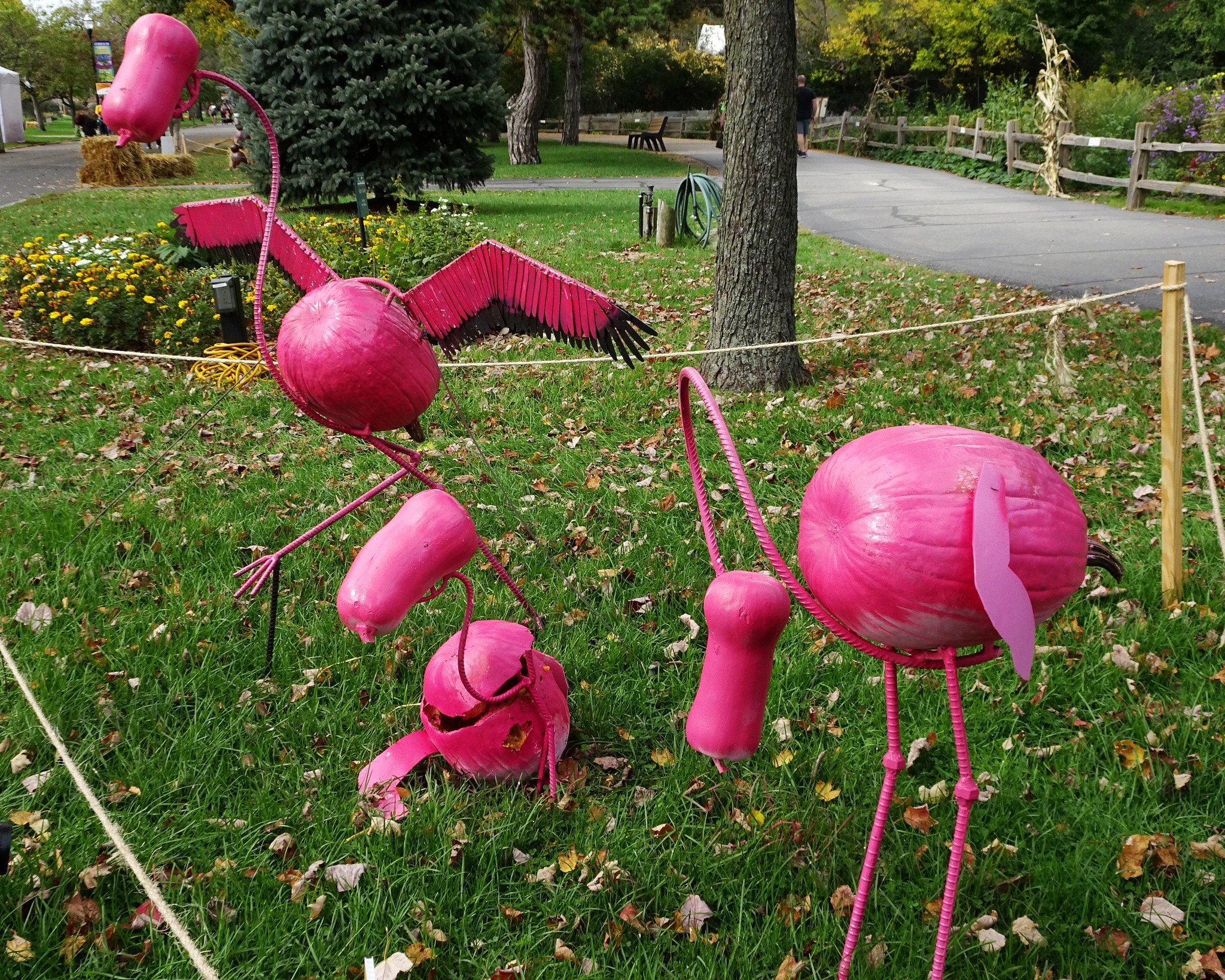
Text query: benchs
628 116 668 152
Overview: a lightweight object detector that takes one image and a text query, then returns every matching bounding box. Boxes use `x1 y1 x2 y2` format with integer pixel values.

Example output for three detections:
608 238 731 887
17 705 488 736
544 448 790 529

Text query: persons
720 101 726 128
83 97 115 135
796 75 817 158
208 99 233 126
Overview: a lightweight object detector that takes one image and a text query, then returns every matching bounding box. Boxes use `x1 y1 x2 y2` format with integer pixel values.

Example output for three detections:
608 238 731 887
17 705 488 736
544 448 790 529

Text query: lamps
212 273 249 344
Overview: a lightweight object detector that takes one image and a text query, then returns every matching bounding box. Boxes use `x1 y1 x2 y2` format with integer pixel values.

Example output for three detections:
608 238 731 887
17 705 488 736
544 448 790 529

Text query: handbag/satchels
715 126 724 149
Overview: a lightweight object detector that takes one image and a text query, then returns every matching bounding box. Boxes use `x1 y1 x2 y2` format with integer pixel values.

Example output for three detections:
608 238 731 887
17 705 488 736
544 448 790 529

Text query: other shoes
799 152 806 158
797 150 802 157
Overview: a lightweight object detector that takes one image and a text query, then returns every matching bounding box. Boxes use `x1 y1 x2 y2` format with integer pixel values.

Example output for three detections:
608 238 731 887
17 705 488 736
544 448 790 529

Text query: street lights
83 15 109 136
